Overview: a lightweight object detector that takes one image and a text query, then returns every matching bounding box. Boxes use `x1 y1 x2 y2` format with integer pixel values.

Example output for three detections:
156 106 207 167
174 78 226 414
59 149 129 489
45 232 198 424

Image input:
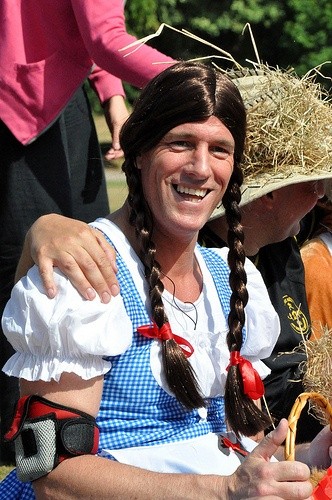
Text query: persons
0 0 182 469
287 183 332 426
1 63 332 500
12 68 332 448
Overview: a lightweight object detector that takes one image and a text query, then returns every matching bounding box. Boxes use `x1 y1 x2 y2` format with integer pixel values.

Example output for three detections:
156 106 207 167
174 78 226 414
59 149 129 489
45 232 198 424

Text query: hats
119 22 332 221
